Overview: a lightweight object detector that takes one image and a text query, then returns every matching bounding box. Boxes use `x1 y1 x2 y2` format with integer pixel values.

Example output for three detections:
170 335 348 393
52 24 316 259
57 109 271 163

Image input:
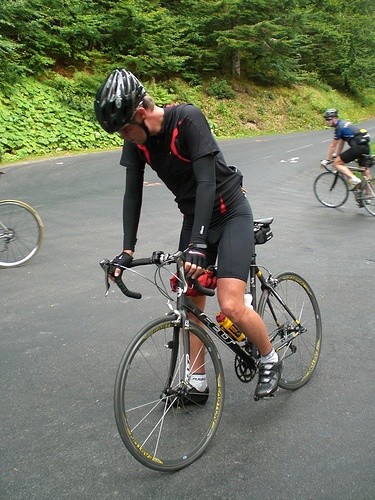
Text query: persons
94 67 283 407
320 108 371 208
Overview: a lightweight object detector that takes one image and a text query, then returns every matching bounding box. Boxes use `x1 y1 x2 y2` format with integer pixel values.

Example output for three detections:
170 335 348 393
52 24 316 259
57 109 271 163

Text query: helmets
94 68 146 135
323 109 338 118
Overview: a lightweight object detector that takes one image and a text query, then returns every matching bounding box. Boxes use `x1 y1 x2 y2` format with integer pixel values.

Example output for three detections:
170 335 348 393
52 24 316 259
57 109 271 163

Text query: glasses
326 118 334 121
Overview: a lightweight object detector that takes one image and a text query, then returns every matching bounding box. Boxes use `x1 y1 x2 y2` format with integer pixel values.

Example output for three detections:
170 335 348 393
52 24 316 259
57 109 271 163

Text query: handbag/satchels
355 133 371 144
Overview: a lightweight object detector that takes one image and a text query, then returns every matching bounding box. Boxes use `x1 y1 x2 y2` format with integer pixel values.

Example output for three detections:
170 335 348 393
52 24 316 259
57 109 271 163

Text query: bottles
215 312 246 341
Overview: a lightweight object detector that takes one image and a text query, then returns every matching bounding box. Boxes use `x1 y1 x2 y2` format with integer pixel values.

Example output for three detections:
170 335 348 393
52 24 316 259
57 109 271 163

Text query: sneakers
357 199 370 208
349 178 361 190
160 380 210 406
254 355 284 397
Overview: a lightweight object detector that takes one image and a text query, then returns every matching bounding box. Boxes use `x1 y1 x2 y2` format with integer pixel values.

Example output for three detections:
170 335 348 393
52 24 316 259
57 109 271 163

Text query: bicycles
0 172 44 269
98 215 323 472
313 154 375 217
